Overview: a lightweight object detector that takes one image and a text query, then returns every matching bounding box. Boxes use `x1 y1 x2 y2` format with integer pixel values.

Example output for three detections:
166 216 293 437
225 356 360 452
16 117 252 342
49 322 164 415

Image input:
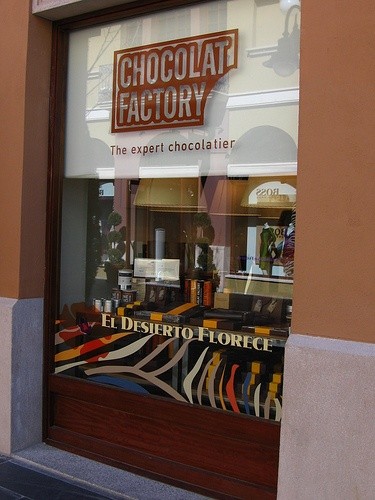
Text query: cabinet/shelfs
71 301 292 422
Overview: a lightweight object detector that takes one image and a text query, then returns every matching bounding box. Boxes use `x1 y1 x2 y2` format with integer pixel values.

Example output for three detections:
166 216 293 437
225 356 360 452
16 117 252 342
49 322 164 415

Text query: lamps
134 178 207 212
241 177 297 209
209 180 261 272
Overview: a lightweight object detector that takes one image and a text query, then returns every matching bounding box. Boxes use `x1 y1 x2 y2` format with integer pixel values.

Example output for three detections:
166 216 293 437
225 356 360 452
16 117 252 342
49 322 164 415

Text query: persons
259 221 276 276
280 223 295 276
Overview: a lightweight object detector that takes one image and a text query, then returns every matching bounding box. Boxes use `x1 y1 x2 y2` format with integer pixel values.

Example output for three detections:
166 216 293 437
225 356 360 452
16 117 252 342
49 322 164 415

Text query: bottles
110 270 137 306
